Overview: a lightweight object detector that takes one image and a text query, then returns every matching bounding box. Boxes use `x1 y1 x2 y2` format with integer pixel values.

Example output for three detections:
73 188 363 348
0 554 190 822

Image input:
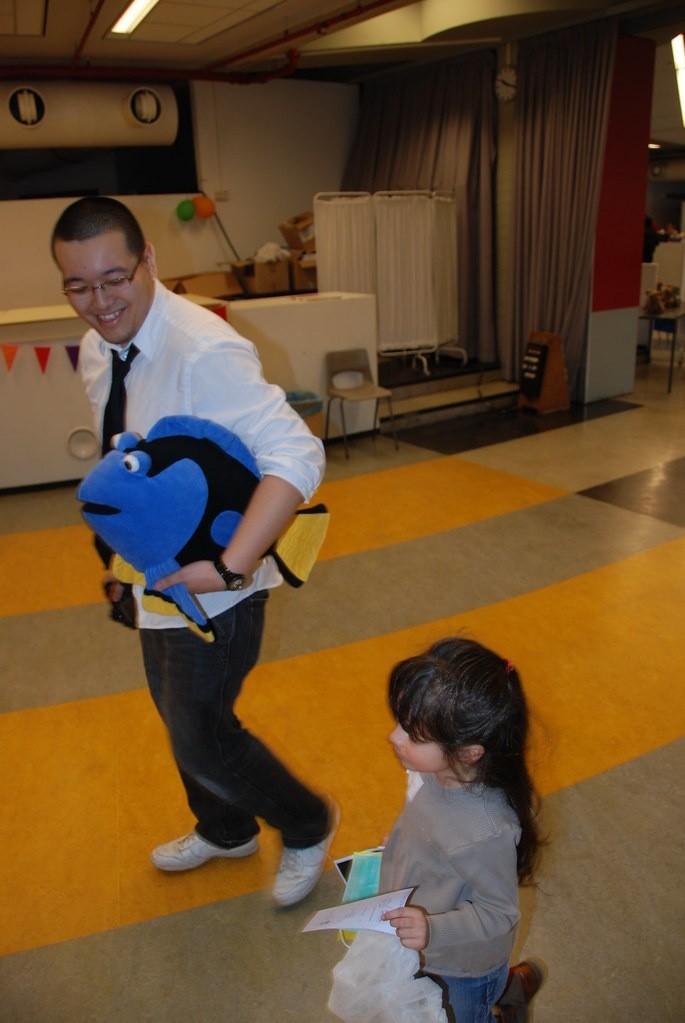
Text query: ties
94 344 141 572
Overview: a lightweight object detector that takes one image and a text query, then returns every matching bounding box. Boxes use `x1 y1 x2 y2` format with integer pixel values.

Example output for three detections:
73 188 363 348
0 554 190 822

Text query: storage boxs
162 212 316 298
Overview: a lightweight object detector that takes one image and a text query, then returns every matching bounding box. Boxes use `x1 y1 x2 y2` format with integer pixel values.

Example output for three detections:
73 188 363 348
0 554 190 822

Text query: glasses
61 249 143 301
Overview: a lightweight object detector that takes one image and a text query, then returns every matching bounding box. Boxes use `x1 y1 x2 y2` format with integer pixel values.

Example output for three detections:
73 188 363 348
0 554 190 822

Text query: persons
48 195 341 906
387 637 543 1023
643 215 659 262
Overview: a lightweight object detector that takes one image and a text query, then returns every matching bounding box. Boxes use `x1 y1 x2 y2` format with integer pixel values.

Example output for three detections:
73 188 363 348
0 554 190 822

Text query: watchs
213 555 246 591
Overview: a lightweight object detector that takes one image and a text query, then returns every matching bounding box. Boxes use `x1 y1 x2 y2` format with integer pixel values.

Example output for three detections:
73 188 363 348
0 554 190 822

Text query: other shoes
495 962 543 1023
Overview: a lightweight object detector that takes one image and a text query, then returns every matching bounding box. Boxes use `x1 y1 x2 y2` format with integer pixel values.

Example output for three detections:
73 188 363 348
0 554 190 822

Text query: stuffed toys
643 281 681 315
76 413 330 643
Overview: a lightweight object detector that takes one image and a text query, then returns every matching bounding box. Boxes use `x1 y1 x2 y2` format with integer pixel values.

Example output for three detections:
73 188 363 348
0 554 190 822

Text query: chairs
324 348 401 461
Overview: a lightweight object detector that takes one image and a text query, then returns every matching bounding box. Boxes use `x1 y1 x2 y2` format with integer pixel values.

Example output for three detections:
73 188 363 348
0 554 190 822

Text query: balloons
193 196 215 218
176 200 195 220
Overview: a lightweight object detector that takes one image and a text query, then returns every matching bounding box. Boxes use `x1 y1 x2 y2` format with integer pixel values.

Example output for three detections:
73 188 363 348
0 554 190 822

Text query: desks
639 306 685 392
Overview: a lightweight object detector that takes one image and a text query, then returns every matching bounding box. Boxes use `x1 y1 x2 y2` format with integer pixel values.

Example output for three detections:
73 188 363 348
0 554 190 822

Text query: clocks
493 65 520 103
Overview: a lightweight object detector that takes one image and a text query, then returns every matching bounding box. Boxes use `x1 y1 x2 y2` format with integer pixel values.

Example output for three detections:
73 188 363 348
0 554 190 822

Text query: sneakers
149 830 261 871
270 792 340 907
